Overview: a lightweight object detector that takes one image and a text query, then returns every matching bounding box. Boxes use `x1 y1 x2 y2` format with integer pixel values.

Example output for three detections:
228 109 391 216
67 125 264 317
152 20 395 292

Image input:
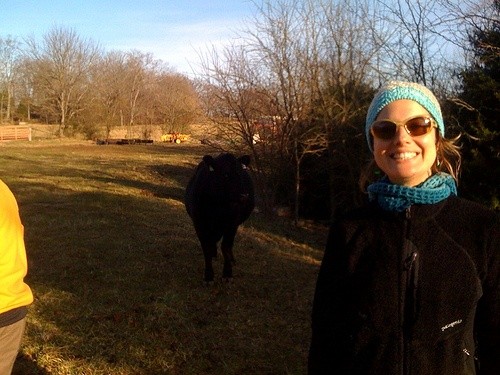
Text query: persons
305 80 500 375
0 181 35 374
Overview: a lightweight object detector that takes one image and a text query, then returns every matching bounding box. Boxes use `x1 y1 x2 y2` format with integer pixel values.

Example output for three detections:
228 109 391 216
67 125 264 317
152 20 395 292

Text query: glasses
369 115 439 141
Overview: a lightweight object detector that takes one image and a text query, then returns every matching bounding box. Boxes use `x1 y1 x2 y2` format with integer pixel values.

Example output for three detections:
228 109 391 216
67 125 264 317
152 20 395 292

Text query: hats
365 80 445 155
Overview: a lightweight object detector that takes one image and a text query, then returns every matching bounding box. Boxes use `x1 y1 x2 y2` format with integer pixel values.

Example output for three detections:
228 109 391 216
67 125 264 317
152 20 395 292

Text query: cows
185 152 255 282
